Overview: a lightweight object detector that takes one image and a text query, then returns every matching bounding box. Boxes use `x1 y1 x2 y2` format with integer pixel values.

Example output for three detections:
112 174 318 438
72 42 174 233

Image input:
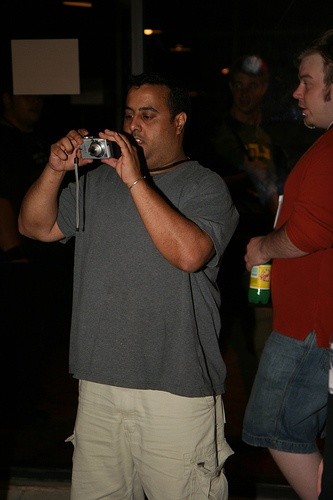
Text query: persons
19 70 240 499
242 33 332 500
0 54 333 454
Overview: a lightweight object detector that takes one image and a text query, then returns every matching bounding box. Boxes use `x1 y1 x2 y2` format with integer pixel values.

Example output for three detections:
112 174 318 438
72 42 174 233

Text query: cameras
80 136 114 159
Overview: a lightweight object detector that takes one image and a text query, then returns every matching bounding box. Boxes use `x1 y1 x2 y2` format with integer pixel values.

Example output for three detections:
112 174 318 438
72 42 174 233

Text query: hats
229 56 268 79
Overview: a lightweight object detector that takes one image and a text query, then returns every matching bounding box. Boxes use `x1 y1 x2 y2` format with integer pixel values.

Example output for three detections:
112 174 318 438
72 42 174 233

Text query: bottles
249 259 272 304
